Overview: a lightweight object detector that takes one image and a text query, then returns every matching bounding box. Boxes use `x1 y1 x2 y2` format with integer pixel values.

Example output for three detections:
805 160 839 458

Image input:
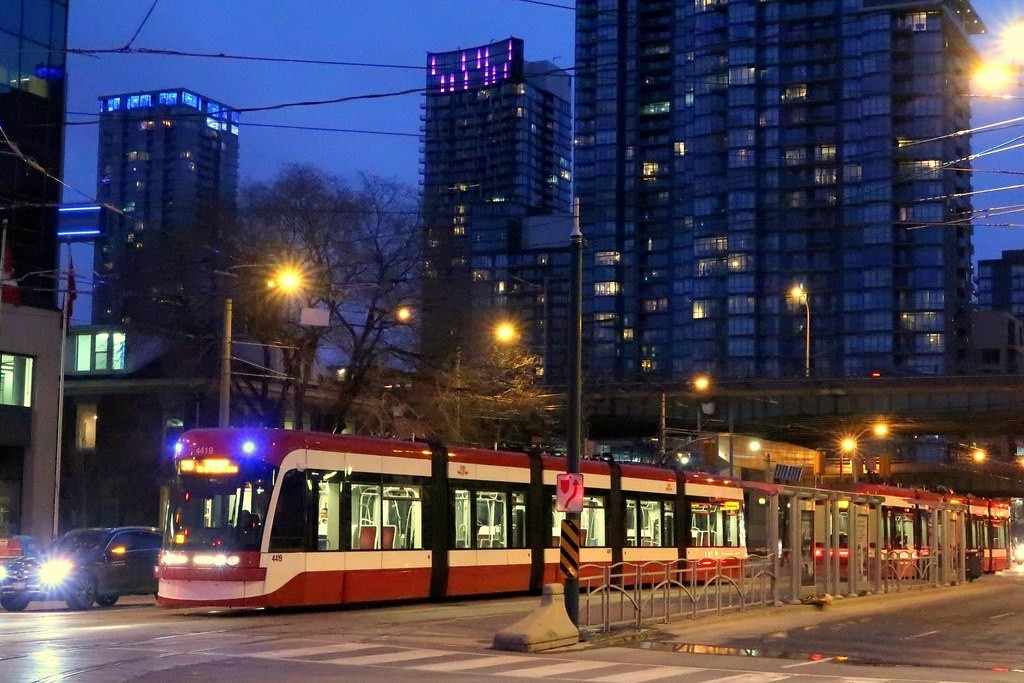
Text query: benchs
553 528 587 547
358 525 402 550
696 531 716 546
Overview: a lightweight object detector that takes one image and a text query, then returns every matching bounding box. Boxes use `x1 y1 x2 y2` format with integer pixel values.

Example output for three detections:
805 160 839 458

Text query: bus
153 427 1011 612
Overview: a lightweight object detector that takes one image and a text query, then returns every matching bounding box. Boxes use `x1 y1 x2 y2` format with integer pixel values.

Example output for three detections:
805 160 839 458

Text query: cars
2 526 159 610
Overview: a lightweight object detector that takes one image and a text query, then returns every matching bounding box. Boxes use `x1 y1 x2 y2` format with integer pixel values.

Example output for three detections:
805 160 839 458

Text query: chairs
477 525 504 549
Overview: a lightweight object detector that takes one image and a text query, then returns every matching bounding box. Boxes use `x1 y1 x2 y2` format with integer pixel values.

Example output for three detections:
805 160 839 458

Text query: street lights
787 279 811 379
452 319 521 435
691 371 715 440
835 422 891 484
218 268 304 429
